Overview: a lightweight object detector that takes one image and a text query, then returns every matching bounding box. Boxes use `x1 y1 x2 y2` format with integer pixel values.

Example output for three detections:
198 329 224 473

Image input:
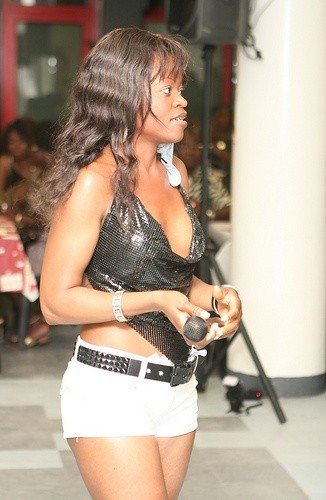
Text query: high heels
10 319 52 348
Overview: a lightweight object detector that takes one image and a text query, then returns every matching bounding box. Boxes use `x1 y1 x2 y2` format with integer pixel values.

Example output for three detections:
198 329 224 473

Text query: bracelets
206 283 238 319
112 289 133 324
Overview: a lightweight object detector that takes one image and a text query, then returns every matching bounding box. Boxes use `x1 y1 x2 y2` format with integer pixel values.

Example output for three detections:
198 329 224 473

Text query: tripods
195 44 286 423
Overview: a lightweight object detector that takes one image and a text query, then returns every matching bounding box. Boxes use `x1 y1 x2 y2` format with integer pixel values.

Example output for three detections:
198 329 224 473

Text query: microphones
182 316 209 342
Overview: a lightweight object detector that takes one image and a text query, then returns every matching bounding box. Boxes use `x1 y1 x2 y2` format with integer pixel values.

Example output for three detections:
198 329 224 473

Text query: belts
72 339 198 388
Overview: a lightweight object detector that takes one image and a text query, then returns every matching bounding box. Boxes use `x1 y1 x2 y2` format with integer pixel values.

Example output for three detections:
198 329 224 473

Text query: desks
0 213 39 352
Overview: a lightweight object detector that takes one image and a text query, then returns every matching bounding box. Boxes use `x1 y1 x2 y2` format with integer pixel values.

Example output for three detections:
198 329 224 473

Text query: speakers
163 0 249 45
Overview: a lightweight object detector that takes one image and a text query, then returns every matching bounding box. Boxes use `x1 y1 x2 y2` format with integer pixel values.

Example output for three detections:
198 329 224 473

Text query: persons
0 113 231 347
33 26 242 500
